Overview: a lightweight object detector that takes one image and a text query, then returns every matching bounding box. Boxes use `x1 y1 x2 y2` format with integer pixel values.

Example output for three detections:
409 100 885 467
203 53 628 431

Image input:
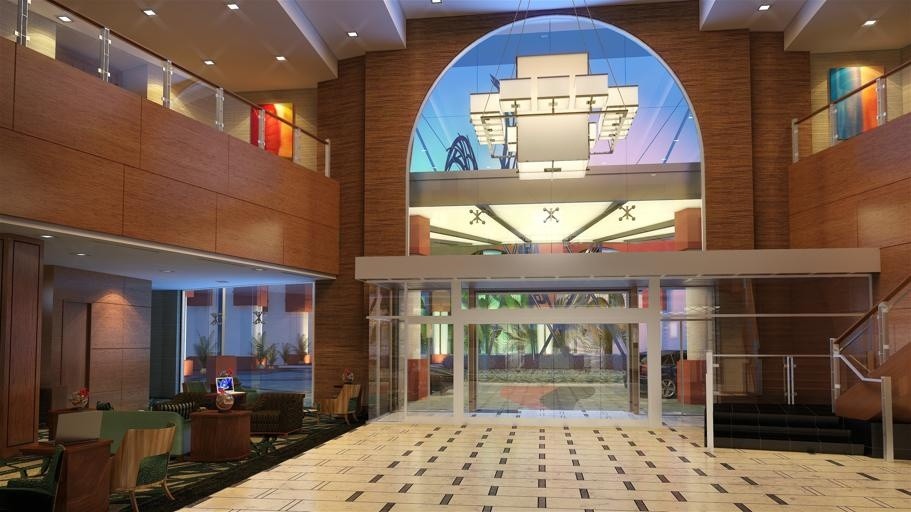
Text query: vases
342 373 353 383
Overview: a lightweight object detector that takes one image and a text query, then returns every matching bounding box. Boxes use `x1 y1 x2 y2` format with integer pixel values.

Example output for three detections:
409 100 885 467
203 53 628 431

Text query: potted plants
192 327 218 375
248 331 268 370
264 343 281 369
290 333 308 364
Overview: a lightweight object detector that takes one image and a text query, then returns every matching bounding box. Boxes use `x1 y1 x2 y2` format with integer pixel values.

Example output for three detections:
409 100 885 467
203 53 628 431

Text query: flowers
343 367 352 376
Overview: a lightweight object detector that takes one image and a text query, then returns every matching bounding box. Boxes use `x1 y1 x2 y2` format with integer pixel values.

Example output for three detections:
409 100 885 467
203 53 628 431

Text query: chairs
239 390 305 436
100 409 183 456
113 423 176 512
151 379 255 419
315 384 361 425
0 445 65 512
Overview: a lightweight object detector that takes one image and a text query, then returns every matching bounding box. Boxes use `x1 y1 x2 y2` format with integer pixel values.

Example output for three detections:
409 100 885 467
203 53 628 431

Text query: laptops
216 377 245 394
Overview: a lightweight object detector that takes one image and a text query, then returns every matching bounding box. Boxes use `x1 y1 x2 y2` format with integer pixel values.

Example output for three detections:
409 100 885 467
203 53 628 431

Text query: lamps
210 311 220 326
469 0 639 181
254 311 266 325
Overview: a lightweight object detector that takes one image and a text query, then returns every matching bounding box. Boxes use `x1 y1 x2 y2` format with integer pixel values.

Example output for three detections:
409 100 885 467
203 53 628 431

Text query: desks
190 408 251 462
49 409 104 439
22 437 113 511
